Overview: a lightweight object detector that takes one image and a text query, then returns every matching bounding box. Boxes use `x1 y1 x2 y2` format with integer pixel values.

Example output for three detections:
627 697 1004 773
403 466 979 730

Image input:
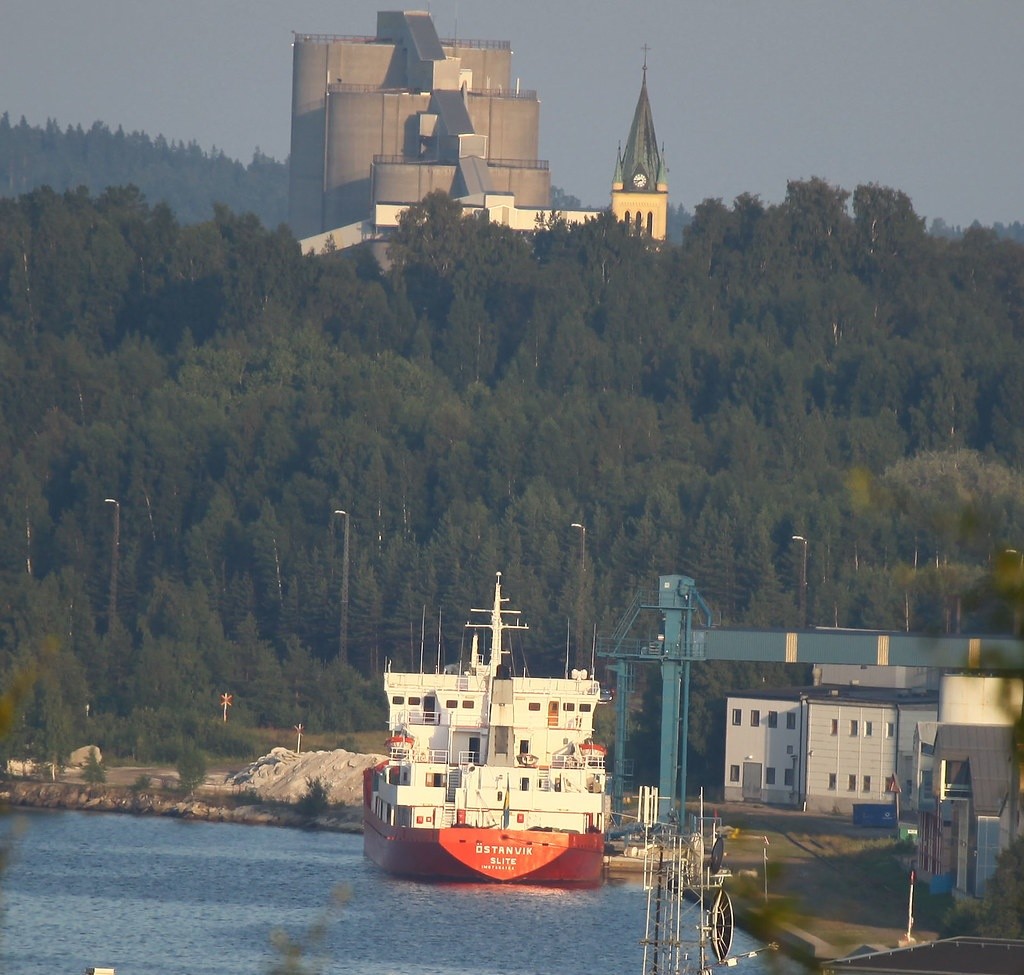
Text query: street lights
792 535 807 630
334 509 348 668
103 498 119 601
1004 549 1024 632
572 523 584 670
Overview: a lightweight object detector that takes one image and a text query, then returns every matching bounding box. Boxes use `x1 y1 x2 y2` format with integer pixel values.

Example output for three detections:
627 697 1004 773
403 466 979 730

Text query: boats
581 744 606 762
385 737 414 757
361 573 607 885
